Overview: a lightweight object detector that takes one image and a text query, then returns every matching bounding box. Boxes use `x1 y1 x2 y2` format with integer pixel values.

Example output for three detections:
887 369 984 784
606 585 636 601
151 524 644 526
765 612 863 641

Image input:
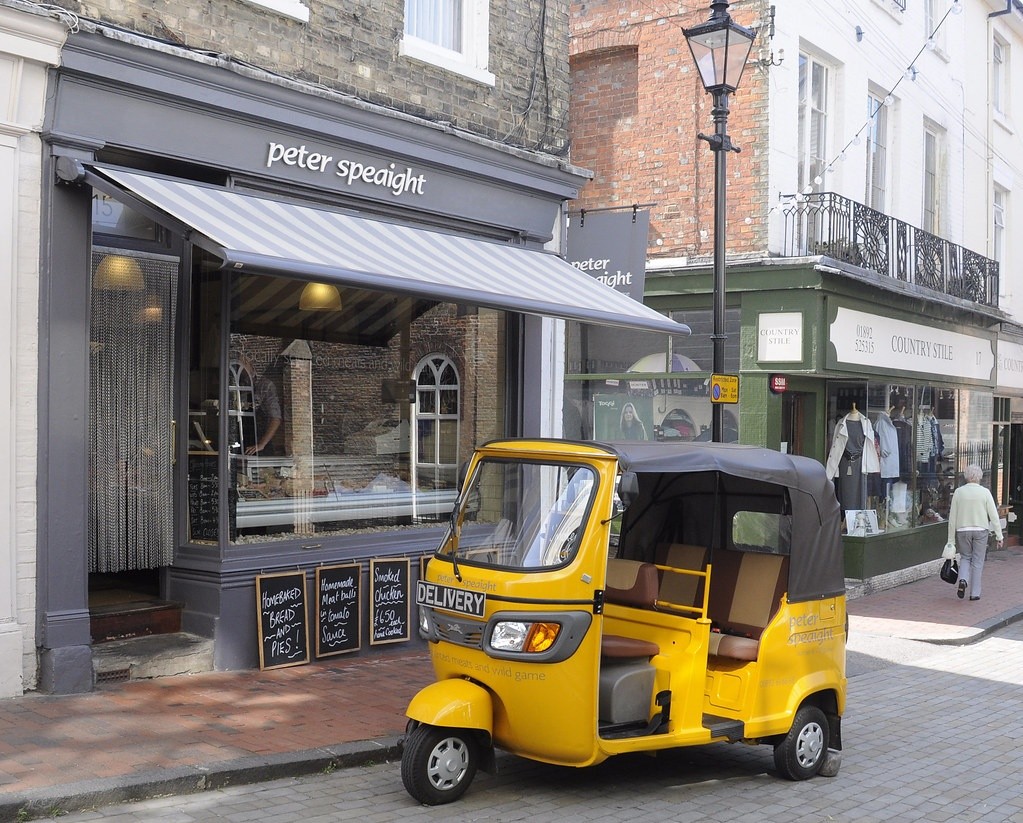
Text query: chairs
601 557 661 663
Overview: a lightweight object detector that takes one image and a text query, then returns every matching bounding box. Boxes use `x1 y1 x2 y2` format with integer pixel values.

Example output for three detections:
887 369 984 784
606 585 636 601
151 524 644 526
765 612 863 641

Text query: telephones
315 564 363 658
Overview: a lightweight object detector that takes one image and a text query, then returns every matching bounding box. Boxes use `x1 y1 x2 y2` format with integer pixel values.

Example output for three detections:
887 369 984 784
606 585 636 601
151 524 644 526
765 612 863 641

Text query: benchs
648 539 790 663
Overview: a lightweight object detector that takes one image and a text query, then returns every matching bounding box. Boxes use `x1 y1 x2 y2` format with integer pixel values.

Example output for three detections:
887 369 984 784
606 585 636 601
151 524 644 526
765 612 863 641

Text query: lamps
93 255 145 293
298 281 342 312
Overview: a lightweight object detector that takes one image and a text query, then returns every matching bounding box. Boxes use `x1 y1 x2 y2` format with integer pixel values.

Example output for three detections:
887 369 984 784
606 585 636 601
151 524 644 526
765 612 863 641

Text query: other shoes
957 579 967 599
969 596 980 601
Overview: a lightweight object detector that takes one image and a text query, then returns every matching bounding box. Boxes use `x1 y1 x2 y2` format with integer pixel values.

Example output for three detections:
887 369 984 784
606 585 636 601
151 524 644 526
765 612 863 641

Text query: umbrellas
625 352 702 413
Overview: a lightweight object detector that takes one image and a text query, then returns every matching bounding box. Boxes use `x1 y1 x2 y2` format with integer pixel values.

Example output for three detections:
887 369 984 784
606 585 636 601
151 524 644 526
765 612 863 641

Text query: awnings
83 164 692 339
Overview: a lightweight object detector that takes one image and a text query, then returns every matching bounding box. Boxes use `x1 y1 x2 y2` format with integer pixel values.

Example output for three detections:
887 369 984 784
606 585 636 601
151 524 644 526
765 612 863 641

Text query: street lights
680 1 758 441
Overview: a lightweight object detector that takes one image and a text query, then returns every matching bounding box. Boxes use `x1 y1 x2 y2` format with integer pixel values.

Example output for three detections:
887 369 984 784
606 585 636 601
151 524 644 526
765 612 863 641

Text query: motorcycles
400 437 850 806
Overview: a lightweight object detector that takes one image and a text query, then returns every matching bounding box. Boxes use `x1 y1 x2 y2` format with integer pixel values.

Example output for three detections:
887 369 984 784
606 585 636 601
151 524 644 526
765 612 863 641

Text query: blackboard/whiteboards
255 571 310 670
238 488 268 501
369 557 411 645
186 451 239 543
420 555 434 582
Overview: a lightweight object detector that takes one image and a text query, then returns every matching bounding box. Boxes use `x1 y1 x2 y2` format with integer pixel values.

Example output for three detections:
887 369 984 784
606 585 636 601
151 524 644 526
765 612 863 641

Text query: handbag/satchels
940 558 959 584
941 543 956 559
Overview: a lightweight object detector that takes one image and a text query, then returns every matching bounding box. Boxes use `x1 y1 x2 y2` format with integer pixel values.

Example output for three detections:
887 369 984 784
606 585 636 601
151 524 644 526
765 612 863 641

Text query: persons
947 465 1004 601
233 349 282 456
836 414 843 424
826 410 944 511
620 402 648 441
